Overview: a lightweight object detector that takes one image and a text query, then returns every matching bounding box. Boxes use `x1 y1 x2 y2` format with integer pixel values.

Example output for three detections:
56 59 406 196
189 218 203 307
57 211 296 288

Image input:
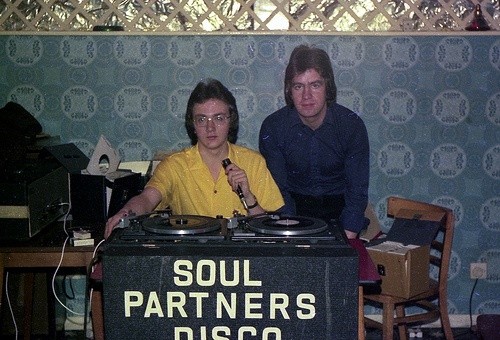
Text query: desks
0 210 360 340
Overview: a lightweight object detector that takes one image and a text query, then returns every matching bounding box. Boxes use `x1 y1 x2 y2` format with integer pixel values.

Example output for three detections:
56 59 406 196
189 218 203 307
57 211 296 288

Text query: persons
88 78 286 339
259 45 370 340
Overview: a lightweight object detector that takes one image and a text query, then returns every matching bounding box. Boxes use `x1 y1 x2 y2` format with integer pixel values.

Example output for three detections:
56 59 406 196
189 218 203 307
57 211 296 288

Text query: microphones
221 158 248 209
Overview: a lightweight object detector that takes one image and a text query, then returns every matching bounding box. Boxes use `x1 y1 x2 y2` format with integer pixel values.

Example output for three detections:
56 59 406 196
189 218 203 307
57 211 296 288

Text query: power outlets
469 262 488 279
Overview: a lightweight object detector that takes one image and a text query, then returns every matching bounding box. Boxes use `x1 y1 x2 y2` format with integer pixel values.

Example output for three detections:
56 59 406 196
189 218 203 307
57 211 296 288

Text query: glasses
191 115 232 127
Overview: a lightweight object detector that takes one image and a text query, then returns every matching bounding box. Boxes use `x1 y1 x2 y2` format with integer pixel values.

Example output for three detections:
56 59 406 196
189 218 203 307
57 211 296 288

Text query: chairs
348 196 455 340
